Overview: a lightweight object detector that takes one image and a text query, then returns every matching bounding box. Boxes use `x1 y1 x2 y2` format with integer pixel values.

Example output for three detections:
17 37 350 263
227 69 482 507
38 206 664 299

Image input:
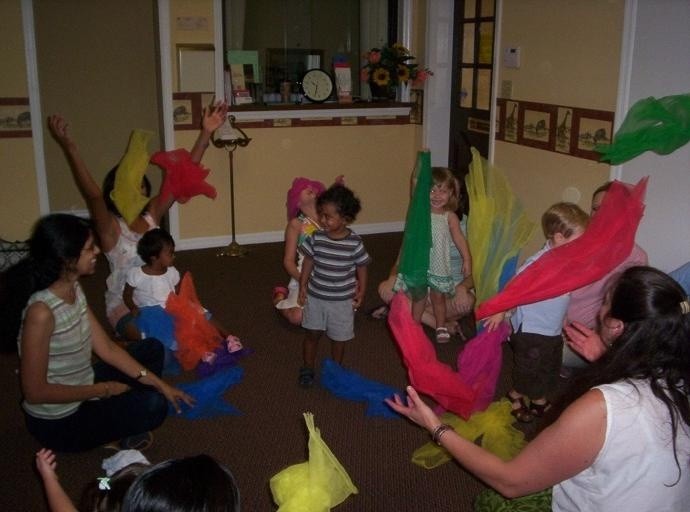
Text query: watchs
132 367 153 388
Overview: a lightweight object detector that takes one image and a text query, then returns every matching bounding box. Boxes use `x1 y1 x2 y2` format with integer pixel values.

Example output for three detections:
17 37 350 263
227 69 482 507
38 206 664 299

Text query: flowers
361 39 436 102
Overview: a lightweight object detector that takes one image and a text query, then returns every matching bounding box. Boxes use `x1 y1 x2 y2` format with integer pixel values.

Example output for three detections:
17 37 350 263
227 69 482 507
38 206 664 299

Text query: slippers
370 303 390 321
300 366 314 386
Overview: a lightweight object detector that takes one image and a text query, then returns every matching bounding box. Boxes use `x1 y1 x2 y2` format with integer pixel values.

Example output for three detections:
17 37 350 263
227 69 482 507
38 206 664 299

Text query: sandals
436 327 451 345
202 354 218 364
220 335 248 353
274 288 285 306
506 393 535 424
526 402 559 418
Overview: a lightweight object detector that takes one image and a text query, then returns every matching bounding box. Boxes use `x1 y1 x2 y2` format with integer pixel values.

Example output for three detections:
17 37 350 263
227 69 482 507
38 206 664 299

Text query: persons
298 186 369 388
480 200 591 424
272 178 326 326
396 149 472 343
123 229 244 363
121 454 241 512
382 266 690 512
35 450 150 512
563 182 648 369
373 178 475 346
49 99 228 344
17 214 197 453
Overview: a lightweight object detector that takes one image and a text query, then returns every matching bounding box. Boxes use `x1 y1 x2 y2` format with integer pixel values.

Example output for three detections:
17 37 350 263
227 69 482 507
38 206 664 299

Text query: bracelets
429 423 456 447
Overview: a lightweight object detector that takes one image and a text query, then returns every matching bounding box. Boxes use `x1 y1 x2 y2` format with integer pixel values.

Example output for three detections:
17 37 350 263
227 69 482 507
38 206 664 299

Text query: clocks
301 69 335 103
